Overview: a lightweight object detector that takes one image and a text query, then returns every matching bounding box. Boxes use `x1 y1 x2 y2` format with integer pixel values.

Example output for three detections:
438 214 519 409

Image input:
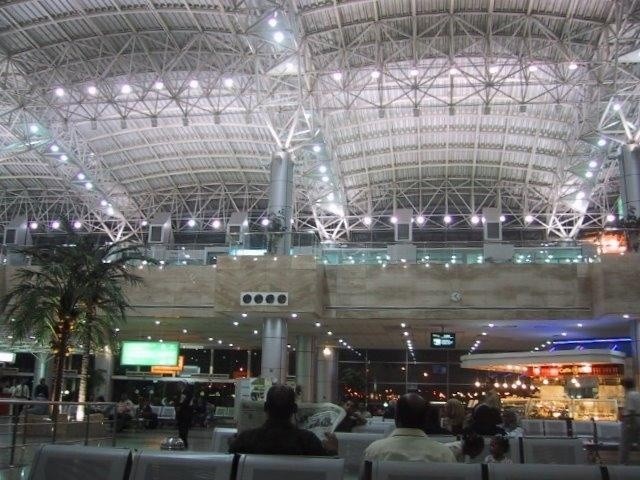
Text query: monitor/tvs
431 332 456 349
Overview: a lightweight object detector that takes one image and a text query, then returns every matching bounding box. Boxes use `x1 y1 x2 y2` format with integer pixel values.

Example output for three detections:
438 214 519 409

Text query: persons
358 392 458 479
426 376 525 464
617 377 640 465
0 376 215 447
228 383 339 458
332 401 367 432
